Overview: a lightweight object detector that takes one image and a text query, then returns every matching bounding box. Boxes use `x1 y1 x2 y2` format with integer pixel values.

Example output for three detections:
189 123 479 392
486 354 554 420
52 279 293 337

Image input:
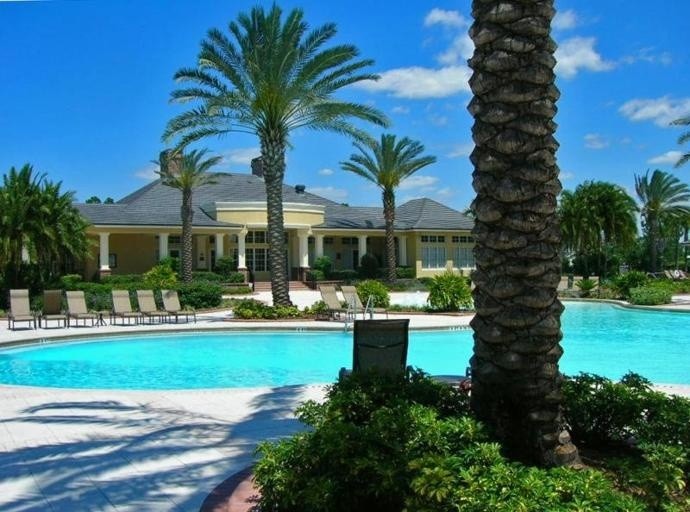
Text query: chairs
7 286 199 331
314 280 418 399
663 267 689 283
